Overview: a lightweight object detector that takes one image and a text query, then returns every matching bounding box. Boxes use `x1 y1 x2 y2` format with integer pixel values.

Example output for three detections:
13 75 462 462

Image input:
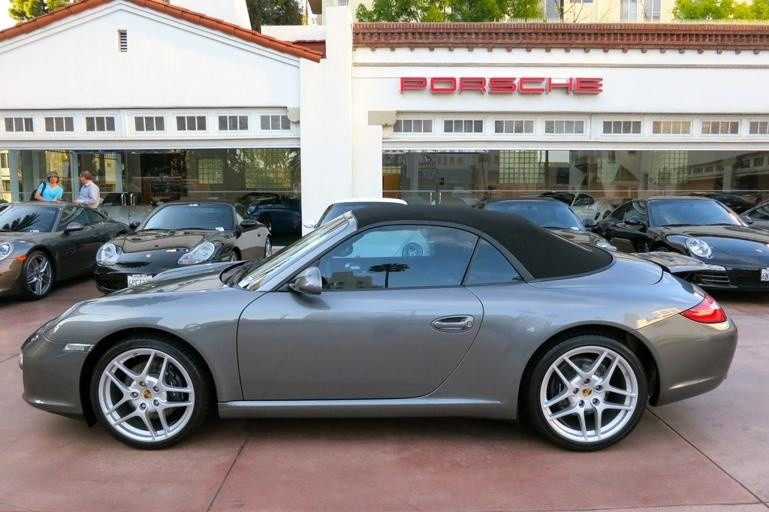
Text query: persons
72 170 101 208
33 170 64 201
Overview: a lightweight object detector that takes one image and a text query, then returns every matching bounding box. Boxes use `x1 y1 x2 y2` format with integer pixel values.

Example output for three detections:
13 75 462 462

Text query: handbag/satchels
29 181 47 201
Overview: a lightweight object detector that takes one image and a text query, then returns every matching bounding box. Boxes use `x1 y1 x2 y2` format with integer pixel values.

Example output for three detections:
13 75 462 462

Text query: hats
46 171 61 179
